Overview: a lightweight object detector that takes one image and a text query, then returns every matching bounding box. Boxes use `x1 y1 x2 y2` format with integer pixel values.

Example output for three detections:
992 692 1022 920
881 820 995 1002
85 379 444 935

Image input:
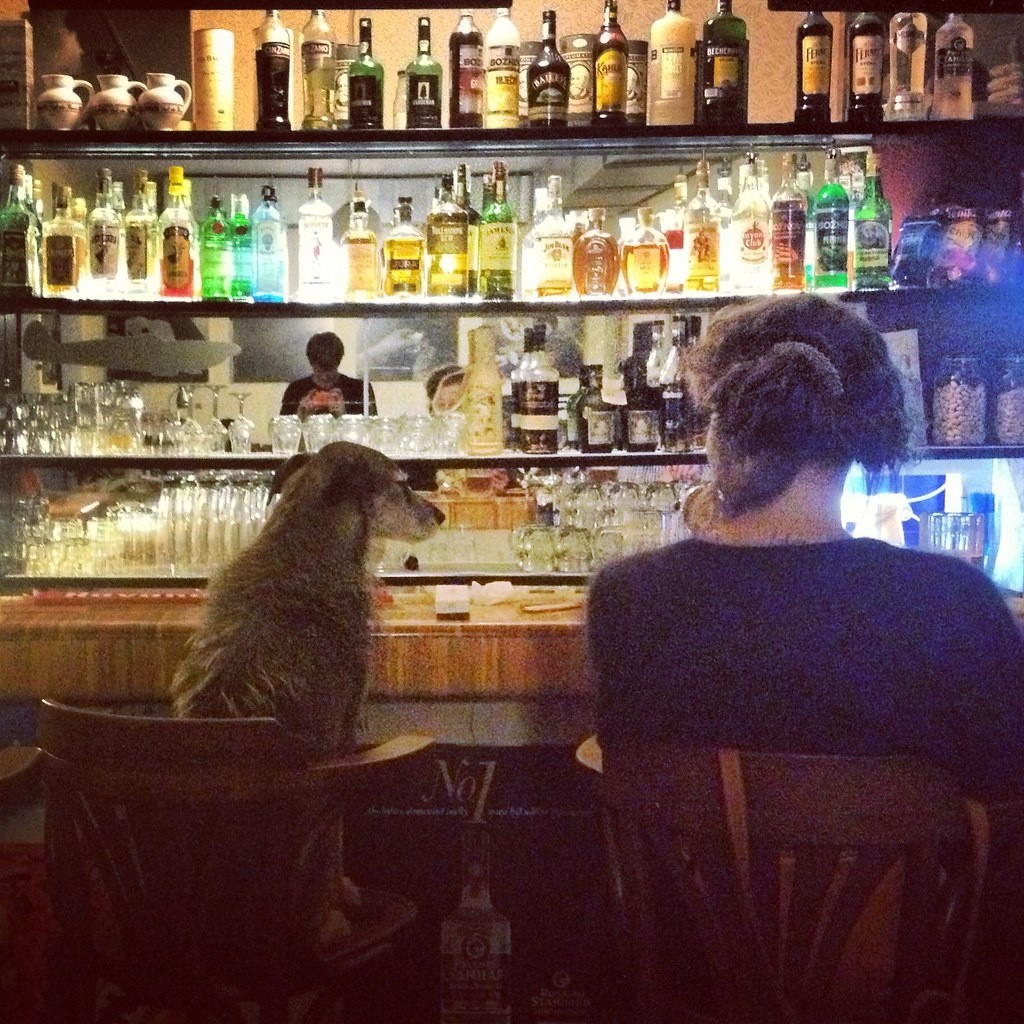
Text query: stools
561 727 1022 1023
34 691 436 1024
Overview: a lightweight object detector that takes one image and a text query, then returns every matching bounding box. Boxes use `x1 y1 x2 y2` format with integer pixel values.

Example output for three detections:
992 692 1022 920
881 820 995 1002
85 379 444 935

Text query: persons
280 333 377 416
589 293 1023 1024
424 365 464 415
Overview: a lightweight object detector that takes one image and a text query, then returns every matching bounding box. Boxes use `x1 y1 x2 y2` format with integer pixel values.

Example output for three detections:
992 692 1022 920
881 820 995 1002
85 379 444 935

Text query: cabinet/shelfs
0 112 1023 463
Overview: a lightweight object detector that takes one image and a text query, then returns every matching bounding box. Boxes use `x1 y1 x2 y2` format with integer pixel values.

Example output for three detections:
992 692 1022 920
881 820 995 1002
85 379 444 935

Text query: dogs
166 441 446 958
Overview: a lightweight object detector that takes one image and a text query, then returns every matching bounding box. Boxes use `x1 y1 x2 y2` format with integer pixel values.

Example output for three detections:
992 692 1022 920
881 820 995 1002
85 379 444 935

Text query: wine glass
179 384 254 453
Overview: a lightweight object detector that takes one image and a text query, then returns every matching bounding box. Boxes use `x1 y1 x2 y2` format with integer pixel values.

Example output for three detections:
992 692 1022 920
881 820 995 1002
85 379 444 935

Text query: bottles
253 0 748 132
795 11 975 124
460 312 708 453
439 829 510 1024
0 146 894 299
931 355 1024 446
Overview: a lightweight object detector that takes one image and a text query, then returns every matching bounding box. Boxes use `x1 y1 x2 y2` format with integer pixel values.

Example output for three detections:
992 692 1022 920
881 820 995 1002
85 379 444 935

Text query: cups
1 468 277 564
0 378 182 456
454 466 692 573
35 73 193 132
194 29 234 133
268 414 463 456
919 512 985 573
